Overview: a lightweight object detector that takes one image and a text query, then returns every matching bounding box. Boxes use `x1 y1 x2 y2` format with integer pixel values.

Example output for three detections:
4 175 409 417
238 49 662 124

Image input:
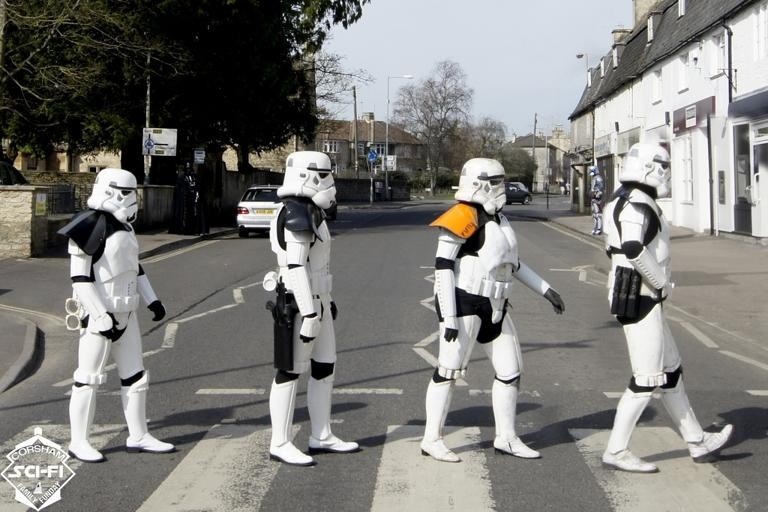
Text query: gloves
95 312 118 340
650 286 667 301
147 301 165 321
299 312 320 342
443 317 458 342
330 302 337 320
543 287 564 314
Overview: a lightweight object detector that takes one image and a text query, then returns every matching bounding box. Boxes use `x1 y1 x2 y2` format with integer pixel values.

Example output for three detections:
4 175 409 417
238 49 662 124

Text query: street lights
385 73 414 200
545 121 564 181
576 50 588 73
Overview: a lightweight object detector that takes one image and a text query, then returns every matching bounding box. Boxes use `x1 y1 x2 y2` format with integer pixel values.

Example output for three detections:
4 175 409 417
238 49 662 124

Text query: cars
236 184 286 237
503 181 533 204
511 181 529 192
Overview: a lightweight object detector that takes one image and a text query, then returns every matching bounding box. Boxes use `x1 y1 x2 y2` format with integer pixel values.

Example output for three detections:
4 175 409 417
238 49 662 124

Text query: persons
585 163 605 235
56 167 176 466
416 157 566 464
260 151 359 467
592 141 735 472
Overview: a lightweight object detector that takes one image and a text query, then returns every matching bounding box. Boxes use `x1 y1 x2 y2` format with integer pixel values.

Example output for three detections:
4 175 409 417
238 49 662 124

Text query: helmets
619 143 669 198
454 156 506 216
276 151 336 209
86 168 138 223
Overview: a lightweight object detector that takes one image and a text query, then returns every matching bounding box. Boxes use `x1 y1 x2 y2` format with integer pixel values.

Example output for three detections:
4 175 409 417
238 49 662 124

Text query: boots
120 371 174 453
269 377 313 467
601 386 658 473
658 374 734 463
420 377 459 462
68 384 103 463
306 374 359 453
491 377 540 459
